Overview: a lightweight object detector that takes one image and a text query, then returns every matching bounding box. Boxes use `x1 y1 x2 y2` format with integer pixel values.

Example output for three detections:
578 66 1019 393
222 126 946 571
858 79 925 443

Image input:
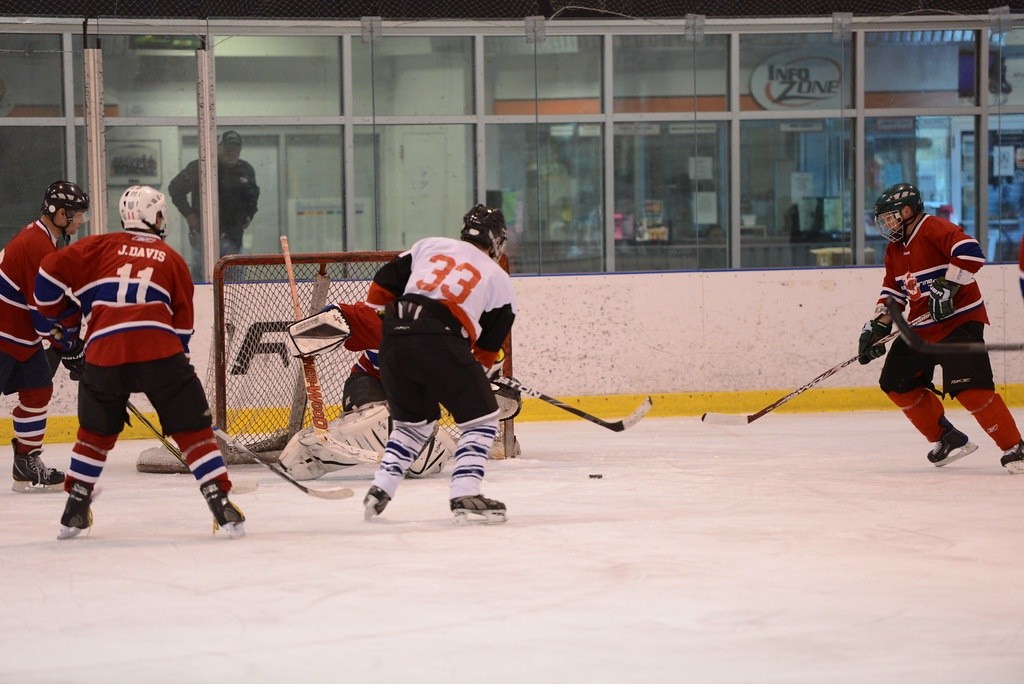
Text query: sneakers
199 479 246 540
363 470 401 522
57 497 93 540
11 437 66 494
1000 439 1024 474
448 477 509 526
927 414 979 467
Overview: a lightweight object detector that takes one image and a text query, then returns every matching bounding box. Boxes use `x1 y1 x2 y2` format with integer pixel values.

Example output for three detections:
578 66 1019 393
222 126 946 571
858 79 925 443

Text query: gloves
62 342 86 381
858 319 894 366
482 358 506 383
929 277 960 322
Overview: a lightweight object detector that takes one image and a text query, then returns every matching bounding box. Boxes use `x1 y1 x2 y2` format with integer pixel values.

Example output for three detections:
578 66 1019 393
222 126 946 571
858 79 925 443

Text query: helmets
119 185 167 230
460 203 508 262
40 181 90 222
874 183 924 242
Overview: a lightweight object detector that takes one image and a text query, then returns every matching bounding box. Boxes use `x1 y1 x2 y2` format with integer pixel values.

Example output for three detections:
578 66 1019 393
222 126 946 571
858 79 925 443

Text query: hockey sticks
886 295 1023 353
210 423 355 501
699 310 931 425
128 400 262 496
498 374 654 434
279 234 384 463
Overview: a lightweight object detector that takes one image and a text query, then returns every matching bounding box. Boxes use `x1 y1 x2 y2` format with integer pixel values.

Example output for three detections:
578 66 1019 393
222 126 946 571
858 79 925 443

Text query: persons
854 184 1024 474
279 204 517 524
33 186 247 541
0 180 91 494
168 130 260 283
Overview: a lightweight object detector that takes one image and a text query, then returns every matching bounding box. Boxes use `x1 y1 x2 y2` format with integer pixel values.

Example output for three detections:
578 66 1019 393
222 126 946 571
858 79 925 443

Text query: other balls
590 474 602 479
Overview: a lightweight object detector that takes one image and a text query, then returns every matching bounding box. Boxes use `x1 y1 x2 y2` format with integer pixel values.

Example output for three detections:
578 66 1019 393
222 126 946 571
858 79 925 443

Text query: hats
218 130 242 146
